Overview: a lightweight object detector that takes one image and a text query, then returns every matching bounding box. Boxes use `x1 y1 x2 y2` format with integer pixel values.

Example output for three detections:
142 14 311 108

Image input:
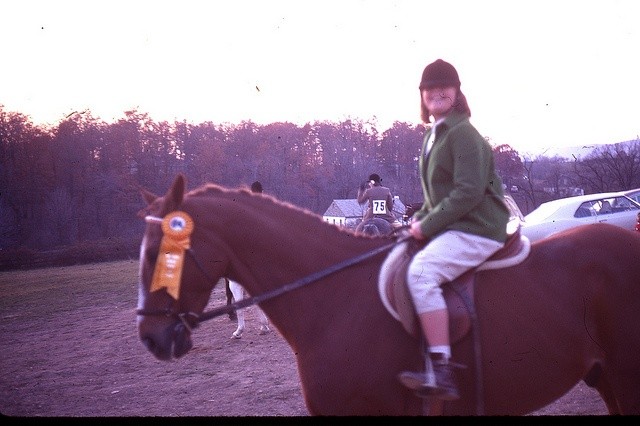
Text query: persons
356 173 402 233
399 59 507 400
252 181 264 195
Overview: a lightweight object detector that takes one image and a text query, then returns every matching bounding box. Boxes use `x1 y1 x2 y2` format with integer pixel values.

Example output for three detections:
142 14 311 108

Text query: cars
522 192 640 244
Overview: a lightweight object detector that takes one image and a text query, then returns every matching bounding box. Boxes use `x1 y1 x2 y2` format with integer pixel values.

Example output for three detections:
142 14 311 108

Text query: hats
252 181 264 193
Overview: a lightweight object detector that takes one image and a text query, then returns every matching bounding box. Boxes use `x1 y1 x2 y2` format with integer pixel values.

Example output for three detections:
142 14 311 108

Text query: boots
398 352 466 402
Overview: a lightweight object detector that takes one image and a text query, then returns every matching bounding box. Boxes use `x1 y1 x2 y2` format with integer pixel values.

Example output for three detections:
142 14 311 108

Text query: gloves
358 181 367 192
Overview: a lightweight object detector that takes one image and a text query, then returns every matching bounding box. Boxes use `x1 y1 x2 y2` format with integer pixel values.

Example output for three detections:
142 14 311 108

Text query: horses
354 217 396 239
227 278 271 340
130 174 640 416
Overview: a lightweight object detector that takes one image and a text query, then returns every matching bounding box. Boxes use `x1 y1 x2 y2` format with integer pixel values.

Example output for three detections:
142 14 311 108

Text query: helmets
418 58 459 87
366 174 381 182
